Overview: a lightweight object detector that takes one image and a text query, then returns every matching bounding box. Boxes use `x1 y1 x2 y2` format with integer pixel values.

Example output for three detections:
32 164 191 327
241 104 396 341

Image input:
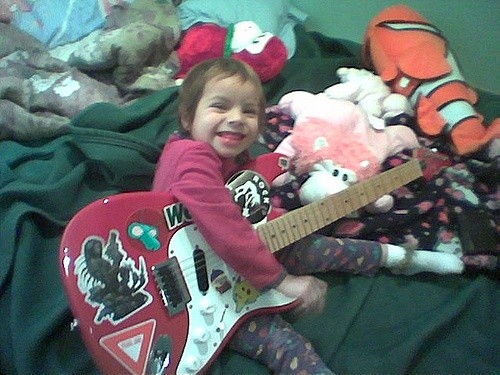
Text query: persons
152 57 466 375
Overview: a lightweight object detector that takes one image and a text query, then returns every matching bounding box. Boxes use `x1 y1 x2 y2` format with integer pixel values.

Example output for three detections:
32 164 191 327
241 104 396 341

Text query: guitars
57 147 458 375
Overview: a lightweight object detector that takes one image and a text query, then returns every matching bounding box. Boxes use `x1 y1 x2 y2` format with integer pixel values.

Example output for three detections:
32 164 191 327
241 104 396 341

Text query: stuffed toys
176 20 288 80
277 90 422 219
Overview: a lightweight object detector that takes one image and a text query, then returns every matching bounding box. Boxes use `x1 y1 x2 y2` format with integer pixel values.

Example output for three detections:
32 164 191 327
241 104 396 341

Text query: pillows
176 0 310 60
